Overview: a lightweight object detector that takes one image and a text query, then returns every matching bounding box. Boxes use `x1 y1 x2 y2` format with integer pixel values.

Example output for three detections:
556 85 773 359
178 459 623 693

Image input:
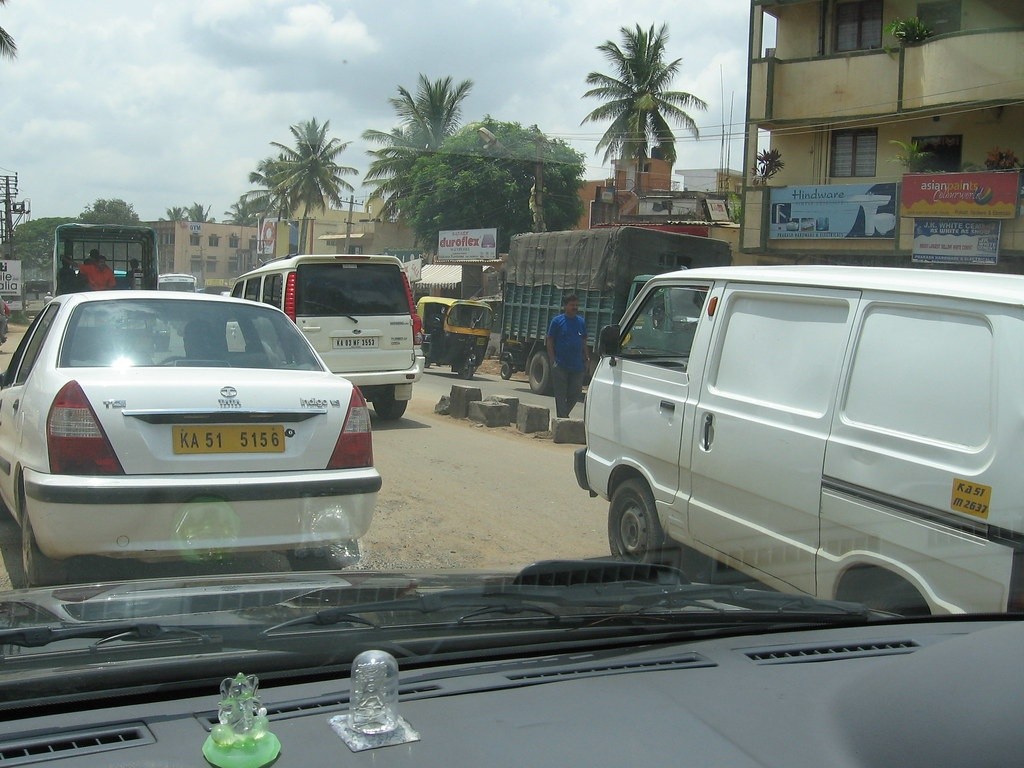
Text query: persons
58 248 117 294
546 294 589 418
182 319 215 359
124 257 140 289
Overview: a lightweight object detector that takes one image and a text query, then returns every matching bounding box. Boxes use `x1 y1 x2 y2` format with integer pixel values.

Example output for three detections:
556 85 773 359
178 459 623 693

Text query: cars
0 556 1023 768
154 318 170 348
25 278 49 293
0 291 382 581
75 269 127 279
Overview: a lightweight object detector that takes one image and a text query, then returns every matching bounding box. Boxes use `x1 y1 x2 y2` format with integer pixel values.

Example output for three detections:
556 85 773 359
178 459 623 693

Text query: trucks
53 222 159 298
500 226 733 395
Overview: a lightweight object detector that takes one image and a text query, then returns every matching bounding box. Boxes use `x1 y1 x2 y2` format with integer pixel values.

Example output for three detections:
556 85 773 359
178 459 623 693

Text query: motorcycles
500 336 544 380
417 296 497 379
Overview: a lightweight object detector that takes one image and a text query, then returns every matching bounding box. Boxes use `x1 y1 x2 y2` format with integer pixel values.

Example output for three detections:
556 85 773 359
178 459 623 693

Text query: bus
159 273 197 293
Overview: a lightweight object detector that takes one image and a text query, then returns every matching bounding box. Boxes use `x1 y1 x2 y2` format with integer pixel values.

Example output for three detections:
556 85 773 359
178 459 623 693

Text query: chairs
182 320 228 363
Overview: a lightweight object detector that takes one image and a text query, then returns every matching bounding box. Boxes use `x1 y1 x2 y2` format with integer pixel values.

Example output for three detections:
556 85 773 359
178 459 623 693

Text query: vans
226 253 426 420
574 263 1024 615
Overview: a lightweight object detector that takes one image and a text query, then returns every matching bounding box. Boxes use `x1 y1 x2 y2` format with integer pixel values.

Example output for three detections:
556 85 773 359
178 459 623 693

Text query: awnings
414 264 490 290
